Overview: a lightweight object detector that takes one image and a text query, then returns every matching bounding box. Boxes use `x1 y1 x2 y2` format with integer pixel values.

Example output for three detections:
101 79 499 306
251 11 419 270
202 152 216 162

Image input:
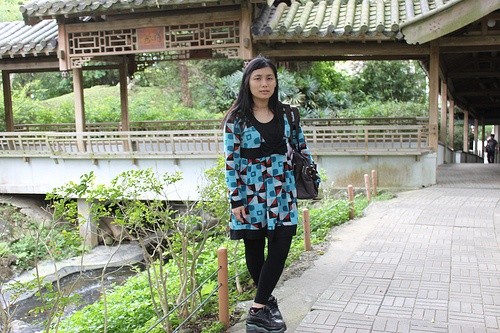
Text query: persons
486 134 498 164
220 57 321 333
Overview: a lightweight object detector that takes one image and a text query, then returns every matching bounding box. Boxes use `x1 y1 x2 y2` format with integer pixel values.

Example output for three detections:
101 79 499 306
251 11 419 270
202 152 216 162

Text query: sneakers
246 297 287 333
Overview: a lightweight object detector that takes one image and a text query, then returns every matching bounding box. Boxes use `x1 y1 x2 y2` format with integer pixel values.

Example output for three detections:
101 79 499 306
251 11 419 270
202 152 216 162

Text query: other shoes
489 160 494 163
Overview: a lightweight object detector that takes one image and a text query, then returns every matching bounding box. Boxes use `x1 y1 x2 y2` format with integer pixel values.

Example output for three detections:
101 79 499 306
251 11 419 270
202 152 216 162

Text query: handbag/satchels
293 153 319 200
